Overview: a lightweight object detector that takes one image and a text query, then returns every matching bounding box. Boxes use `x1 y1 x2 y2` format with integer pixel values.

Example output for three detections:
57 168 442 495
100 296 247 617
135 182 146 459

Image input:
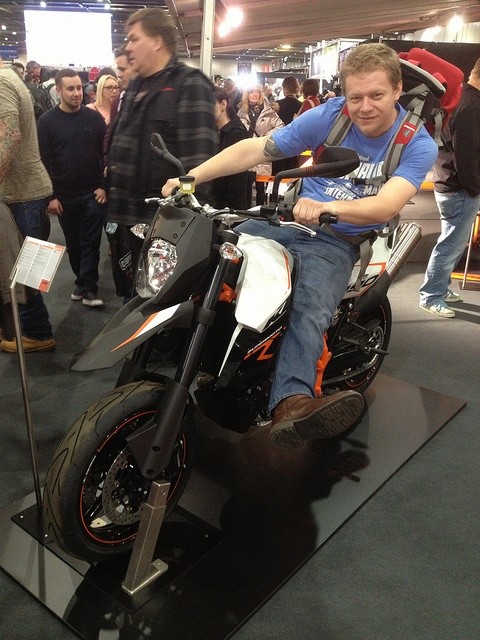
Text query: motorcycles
42 131 424 562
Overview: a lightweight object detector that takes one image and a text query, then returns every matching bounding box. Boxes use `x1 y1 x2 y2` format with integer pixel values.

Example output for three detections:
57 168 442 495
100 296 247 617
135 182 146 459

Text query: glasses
104 84 121 92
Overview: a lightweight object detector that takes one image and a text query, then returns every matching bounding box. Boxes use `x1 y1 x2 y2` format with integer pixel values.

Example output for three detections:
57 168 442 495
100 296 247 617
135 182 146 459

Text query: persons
213 74 224 87
272 76 303 176
212 85 249 209
292 79 321 120
1 53 55 355
11 43 141 107
37 68 107 308
223 79 242 112
102 6 218 307
236 82 286 209
418 57 480 320
161 42 439 445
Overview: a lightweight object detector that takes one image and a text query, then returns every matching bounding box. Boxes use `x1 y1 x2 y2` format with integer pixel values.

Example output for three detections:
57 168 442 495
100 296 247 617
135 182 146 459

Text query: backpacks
280 46 465 293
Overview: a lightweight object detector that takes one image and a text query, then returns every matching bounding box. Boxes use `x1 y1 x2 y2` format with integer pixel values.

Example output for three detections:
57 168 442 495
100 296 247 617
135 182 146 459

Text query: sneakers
421 296 456 320
0 336 59 354
82 294 103 308
71 287 83 300
441 288 462 303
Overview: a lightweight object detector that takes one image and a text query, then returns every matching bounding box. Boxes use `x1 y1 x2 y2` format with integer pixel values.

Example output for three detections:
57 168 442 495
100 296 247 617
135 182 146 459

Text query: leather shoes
267 390 366 451
